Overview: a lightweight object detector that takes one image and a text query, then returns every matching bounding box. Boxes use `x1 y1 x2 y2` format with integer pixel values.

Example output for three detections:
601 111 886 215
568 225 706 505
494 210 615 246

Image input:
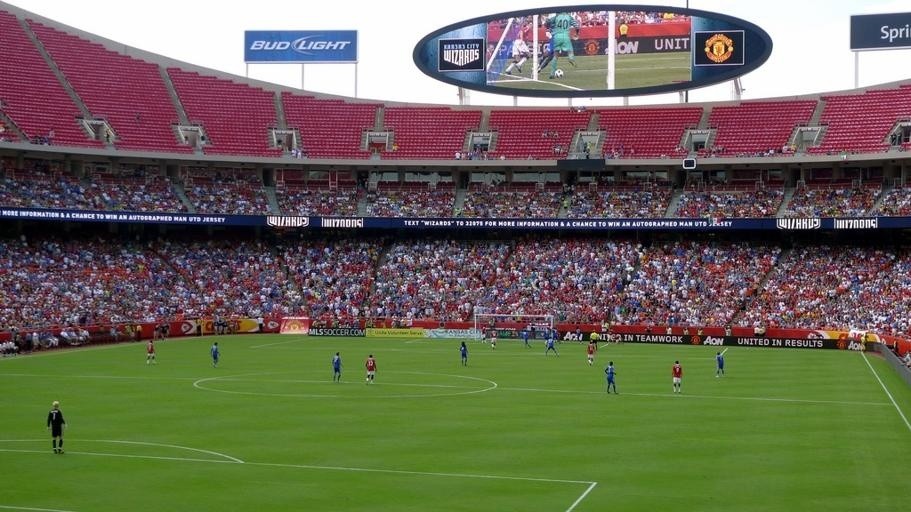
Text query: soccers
556 69 564 78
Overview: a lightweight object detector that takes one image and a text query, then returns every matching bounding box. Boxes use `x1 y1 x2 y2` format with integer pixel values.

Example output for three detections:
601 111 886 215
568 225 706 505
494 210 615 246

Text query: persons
486 10 692 75
604 143 637 159
332 352 345 383
672 360 682 392
0 161 911 220
541 129 558 138
715 351 724 377
46 399 66 454
826 146 849 160
896 146 904 153
605 361 616 392
528 154 540 160
1 233 910 367
292 149 302 158
500 153 505 160
888 130 902 145
392 143 401 151
552 143 568 155
660 143 795 159
31 136 52 146
454 147 489 160
365 355 377 385
580 138 593 153
